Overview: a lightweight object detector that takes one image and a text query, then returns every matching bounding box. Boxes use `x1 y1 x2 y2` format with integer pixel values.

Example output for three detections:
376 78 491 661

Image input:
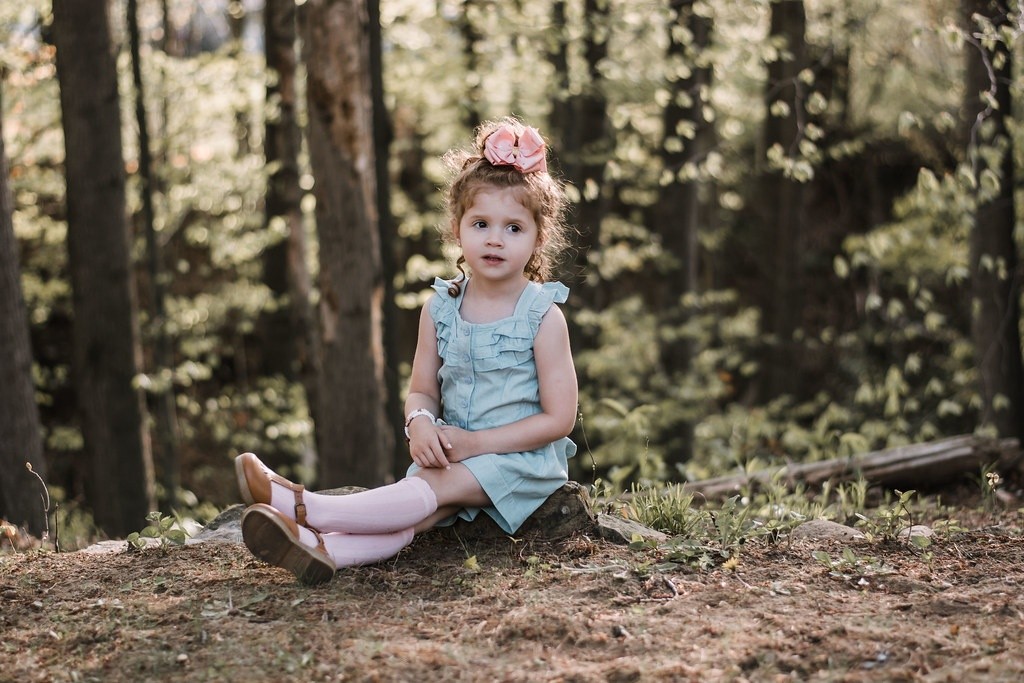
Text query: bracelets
404 409 435 439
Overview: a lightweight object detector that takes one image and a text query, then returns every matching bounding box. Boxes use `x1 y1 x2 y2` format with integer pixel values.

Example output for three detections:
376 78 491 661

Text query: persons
235 121 577 588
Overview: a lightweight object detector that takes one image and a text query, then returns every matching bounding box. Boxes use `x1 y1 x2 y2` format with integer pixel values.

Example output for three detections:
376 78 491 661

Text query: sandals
241 501 337 588
234 451 321 532
